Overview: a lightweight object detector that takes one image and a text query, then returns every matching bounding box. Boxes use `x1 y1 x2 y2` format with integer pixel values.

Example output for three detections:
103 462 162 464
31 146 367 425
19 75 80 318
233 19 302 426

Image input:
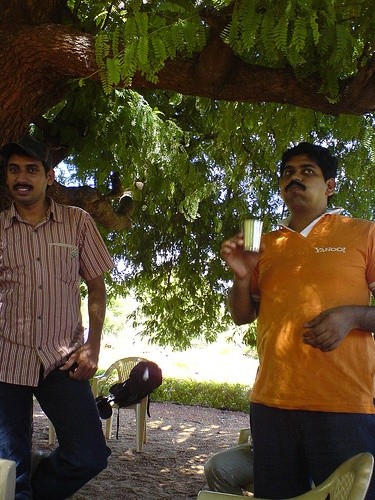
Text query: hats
0 136 52 170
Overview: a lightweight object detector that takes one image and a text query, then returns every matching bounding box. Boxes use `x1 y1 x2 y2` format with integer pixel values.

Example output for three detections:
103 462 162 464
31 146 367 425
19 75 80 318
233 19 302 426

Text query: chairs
196 452 374 500
92 356 150 454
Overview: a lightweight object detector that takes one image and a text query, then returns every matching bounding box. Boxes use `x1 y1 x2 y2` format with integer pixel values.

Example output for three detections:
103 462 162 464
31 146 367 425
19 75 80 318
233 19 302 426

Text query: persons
204 442 254 495
0 136 114 500
220 143 375 500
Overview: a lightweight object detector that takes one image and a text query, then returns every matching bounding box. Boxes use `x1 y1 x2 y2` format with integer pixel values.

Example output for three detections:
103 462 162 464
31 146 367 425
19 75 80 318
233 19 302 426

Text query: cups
239 218 264 253
